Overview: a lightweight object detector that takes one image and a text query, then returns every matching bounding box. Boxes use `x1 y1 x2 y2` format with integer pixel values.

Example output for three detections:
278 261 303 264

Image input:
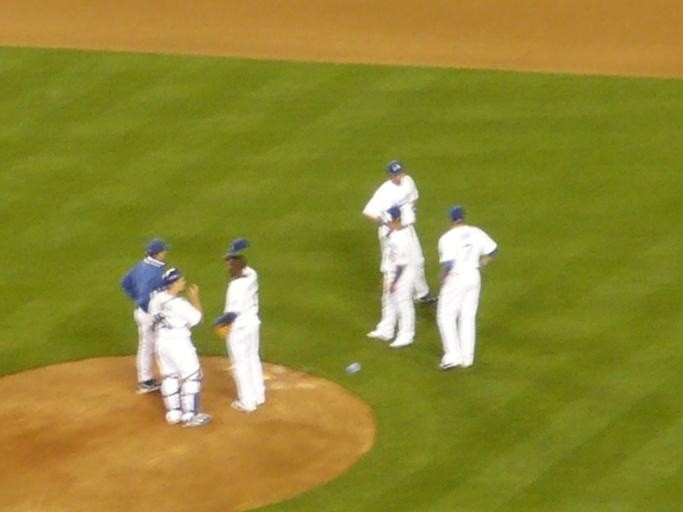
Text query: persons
147 266 211 428
215 239 265 413
434 205 498 369
361 160 436 303
120 239 169 395
365 207 419 347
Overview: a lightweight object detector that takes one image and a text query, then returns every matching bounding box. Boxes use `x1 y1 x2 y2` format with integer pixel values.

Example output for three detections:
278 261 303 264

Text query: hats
388 207 401 218
450 207 463 220
147 238 166 256
157 267 182 292
389 162 403 176
231 238 249 251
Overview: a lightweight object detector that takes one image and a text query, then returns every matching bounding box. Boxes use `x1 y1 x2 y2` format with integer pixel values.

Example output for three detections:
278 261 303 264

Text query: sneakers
229 397 265 413
136 380 161 395
439 359 458 370
366 329 414 348
182 412 211 427
419 295 439 305
